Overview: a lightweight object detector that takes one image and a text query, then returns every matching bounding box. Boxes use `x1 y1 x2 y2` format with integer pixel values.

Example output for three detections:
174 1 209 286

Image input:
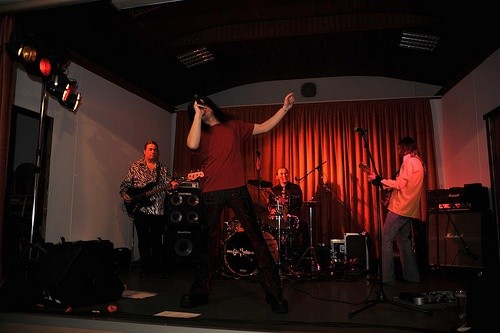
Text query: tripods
292 206 325 283
348 134 435 319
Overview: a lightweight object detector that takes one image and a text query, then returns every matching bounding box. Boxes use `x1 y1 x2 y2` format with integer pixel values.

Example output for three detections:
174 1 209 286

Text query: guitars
124 168 205 216
359 162 395 207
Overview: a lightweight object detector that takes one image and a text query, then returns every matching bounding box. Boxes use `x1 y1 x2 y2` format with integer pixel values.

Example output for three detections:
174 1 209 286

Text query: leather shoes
265 290 289 314
181 289 210 308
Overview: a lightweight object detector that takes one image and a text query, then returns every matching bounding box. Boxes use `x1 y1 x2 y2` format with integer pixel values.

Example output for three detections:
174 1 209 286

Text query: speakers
164 187 209 274
426 210 496 272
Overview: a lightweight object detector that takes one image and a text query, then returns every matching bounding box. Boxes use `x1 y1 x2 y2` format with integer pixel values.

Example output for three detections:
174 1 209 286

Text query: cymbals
302 201 320 206
248 179 273 187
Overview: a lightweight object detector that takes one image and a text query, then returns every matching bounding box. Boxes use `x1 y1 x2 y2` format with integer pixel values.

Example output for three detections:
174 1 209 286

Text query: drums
223 231 279 276
268 208 288 229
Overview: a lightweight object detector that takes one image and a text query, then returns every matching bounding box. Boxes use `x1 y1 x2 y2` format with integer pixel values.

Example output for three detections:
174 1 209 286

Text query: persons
268 167 310 255
119 140 179 279
367 136 428 286
180 93 295 315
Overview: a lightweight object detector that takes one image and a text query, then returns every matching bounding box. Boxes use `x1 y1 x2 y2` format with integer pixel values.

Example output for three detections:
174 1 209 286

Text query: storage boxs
344 233 369 273
330 239 344 270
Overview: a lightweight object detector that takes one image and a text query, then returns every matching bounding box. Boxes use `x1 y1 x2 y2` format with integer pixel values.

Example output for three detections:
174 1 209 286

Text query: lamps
6 32 81 112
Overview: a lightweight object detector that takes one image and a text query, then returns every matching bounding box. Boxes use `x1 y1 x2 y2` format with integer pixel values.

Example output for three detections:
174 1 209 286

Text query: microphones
354 128 368 132
194 94 203 110
295 177 300 181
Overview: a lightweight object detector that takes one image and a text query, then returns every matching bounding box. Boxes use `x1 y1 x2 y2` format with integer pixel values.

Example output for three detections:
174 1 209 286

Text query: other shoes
375 279 385 285
